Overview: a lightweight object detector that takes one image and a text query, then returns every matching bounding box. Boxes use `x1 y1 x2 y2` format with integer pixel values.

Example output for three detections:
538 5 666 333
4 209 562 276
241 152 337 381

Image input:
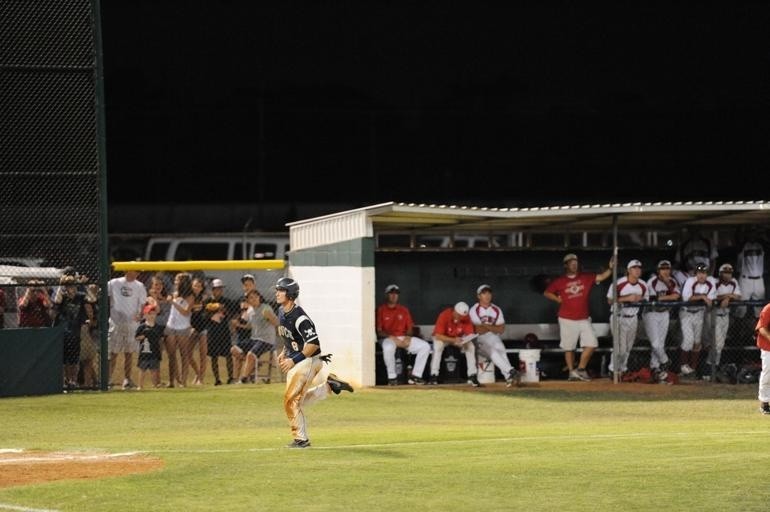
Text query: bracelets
292 351 306 364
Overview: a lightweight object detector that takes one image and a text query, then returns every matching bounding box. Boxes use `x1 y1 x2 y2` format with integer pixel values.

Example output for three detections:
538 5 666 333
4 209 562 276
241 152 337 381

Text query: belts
649 308 668 312
617 314 634 318
682 308 704 313
716 312 727 316
743 276 760 279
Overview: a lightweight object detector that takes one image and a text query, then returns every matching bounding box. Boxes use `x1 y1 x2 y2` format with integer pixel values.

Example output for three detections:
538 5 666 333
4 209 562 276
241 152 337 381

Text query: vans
140 234 289 313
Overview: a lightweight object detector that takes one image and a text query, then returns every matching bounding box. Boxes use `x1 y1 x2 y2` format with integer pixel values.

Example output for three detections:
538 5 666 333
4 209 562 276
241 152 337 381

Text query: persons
754 303 770 414
427 301 481 387
543 253 614 382
468 284 520 388
375 284 431 386
605 225 770 384
0 270 280 395
273 277 353 448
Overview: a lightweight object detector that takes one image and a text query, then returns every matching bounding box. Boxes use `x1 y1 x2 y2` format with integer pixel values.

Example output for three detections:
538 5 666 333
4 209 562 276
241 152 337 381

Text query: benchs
377 340 763 385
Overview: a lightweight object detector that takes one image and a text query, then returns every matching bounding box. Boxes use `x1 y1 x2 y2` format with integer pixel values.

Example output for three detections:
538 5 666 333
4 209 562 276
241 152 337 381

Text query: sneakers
123 375 255 390
661 359 672 370
389 378 400 385
760 405 770 415
568 367 592 381
408 376 426 385
285 439 310 448
467 374 479 387
428 376 437 385
651 368 667 380
327 373 353 394
681 363 695 374
505 369 520 387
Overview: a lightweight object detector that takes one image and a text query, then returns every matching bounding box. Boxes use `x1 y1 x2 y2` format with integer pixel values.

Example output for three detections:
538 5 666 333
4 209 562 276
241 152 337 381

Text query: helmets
275 278 299 298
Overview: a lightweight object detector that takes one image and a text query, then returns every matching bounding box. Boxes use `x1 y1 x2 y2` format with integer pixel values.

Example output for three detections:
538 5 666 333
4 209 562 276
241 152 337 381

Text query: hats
696 263 708 272
477 284 491 298
143 303 157 313
627 260 642 269
719 263 734 272
63 266 75 280
454 302 469 316
212 279 224 288
564 254 577 263
242 274 254 284
386 285 400 294
658 260 671 268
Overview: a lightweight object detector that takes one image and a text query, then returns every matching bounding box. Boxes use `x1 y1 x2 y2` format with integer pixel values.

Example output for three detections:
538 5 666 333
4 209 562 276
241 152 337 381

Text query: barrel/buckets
518 349 542 383
